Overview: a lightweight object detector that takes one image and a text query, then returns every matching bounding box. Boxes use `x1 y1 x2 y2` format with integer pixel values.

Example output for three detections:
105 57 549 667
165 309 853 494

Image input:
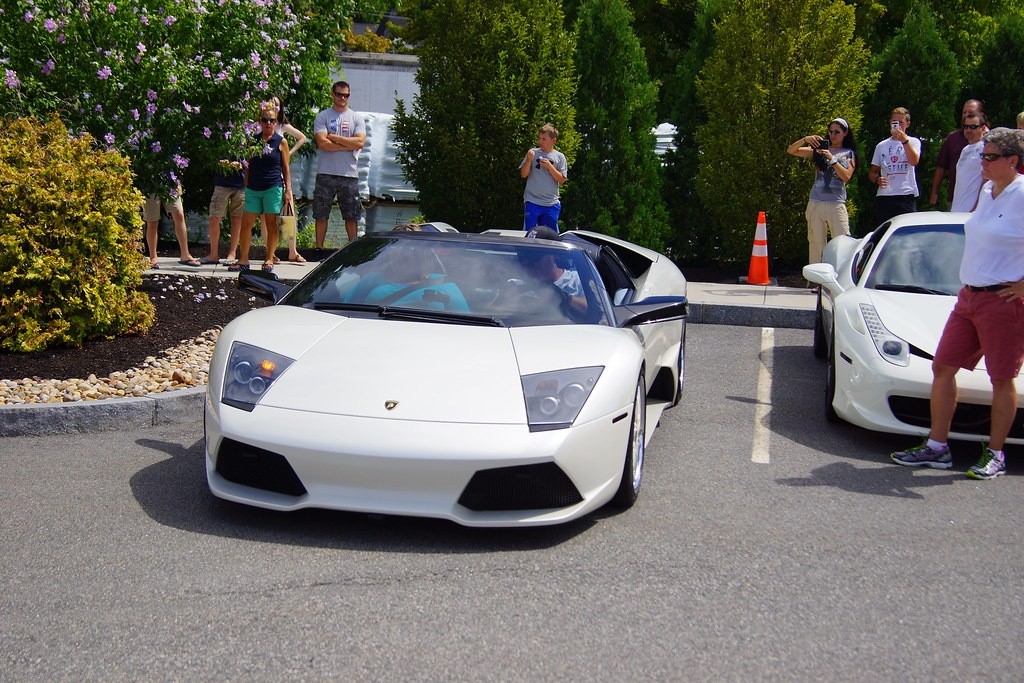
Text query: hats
518 225 560 254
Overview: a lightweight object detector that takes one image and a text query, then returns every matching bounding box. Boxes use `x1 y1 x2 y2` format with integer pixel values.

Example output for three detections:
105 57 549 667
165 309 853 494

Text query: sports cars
802 210 1023 448
203 221 690 526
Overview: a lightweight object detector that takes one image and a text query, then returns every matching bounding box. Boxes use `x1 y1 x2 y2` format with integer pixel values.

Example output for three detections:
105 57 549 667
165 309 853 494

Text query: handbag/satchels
277 202 296 242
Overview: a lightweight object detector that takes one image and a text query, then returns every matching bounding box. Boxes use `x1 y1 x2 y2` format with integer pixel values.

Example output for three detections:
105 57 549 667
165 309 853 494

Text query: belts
964 283 1010 292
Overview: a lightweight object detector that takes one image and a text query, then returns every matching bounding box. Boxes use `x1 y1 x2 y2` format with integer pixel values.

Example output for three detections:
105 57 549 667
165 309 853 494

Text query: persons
140 177 202 269
518 124 567 234
253 96 307 264
490 226 603 324
228 102 292 271
787 118 859 293
928 99 992 211
306 81 366 262
1017 112 1024 130
197 160 245 265
868 107 921 222
341 221 472 315
949 113 990 212
890 127 1024 479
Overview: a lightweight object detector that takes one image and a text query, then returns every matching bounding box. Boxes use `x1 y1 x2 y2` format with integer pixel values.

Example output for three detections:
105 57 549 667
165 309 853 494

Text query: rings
822 152 823 154
1009 291 1014 294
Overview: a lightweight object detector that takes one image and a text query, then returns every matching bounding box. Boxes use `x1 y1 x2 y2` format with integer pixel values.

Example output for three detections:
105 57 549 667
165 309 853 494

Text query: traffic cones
740 212 778 287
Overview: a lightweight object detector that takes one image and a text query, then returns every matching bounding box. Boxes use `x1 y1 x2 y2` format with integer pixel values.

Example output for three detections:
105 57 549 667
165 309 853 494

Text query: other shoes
811 286 818 293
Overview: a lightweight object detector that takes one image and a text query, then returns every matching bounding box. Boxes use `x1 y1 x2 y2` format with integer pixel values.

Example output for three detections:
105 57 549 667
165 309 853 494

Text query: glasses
829 130 841 135
980 152 1006 162
963 124 981 129
516 255 542 262
260 118 277 124
332 89 350 98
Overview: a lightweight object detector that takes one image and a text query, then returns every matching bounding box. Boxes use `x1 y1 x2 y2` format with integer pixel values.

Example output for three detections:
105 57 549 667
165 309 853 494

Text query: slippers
223 260 237 266
228 263 250 271
197 257 220 264
289 254 306 263
262 261 273 272
178 259 200 266
150 262 159 269
272 255 280 264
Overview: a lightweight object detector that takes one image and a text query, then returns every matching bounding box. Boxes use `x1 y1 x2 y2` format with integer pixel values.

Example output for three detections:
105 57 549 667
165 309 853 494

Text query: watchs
829 156 837 165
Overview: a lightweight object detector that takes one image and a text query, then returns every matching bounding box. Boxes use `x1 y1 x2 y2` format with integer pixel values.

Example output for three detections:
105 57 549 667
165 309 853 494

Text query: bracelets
902 139 909 145
875 176 879 184
804 138 808 143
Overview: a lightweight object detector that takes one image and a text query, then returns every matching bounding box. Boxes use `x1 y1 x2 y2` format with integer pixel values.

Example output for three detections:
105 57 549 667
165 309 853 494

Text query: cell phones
811 140 828 149
892 122 900 130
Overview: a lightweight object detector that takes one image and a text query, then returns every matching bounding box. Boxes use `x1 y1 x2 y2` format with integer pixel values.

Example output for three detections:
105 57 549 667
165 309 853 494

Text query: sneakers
890 432 952 469
966 438 1007 480
309 248 322 262
